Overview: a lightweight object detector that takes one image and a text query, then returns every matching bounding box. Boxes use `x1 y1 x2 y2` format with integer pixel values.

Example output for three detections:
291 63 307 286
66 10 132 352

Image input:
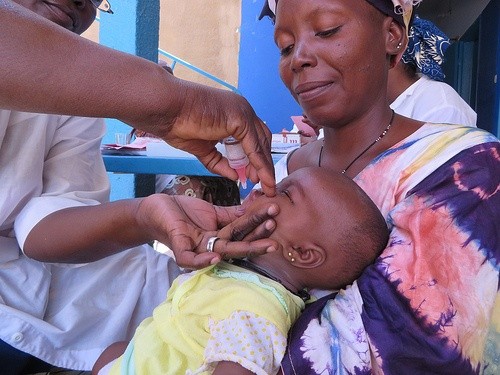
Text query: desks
102 154 291 200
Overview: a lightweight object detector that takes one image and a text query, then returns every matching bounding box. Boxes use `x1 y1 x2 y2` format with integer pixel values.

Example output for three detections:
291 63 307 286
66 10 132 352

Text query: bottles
225 137 250 190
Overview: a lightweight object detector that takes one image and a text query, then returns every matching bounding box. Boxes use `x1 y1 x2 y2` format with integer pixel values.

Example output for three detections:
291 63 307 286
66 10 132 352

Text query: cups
116 133 131 146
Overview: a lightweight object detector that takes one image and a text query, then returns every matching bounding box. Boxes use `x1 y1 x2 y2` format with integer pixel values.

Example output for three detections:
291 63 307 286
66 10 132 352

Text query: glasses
90 0 114 15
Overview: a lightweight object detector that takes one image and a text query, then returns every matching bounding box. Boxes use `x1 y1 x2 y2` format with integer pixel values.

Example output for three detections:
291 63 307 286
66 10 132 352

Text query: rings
207 236 221 253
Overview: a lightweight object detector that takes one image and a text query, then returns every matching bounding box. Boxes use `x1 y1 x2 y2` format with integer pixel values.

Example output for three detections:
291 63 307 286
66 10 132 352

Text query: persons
298 20 477 147
98 166 390 375
244 1 500 375
0 0 281 375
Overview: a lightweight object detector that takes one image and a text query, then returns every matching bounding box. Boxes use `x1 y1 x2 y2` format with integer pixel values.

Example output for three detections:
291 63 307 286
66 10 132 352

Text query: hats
400 13 450 81
259 0 422 70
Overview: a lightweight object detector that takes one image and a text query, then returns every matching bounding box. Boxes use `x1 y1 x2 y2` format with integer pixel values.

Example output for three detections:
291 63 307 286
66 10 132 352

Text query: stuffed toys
160 175 242 207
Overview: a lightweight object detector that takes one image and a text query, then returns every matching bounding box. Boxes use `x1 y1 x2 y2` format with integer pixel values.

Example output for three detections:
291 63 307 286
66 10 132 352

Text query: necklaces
319 108 395 176
227 258 311 302
